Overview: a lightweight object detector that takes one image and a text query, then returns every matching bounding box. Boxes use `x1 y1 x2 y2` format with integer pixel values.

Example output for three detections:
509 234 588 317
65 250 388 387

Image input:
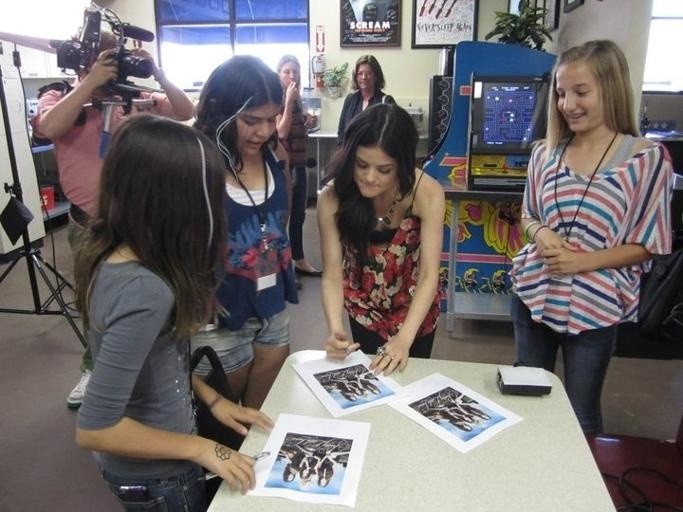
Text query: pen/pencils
197 452 270 485
337 336 350 354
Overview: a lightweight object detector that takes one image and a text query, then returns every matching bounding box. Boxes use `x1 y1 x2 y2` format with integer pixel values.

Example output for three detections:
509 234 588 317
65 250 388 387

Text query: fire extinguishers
312 53 325 89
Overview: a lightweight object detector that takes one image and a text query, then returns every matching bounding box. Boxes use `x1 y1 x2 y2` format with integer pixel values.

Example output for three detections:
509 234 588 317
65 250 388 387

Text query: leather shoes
294 263 323 277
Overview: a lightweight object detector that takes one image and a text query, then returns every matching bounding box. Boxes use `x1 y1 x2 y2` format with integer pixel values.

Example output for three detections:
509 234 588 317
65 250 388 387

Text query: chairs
185 344 243 505
586 417 682 510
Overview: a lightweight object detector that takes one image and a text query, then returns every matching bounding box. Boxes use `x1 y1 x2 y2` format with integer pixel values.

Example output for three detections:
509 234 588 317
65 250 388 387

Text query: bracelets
206 393 225 411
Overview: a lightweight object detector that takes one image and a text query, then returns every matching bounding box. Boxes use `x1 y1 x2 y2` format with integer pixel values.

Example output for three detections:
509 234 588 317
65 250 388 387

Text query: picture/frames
338 1 403 51
541 0 560 33
410 0 479 51
563 0 585 15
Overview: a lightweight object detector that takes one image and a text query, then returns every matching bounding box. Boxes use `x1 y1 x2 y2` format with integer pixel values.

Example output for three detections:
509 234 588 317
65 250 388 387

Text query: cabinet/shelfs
21 75 76 223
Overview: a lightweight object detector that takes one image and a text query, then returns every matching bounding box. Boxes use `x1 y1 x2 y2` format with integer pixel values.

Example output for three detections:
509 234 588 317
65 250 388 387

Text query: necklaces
373 189 403 223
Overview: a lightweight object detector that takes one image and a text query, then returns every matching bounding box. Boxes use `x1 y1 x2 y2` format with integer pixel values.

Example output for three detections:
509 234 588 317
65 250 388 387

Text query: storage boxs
39 182 55 211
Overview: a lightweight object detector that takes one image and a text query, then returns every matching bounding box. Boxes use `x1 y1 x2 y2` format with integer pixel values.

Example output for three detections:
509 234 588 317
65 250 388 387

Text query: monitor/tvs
470 73 556 154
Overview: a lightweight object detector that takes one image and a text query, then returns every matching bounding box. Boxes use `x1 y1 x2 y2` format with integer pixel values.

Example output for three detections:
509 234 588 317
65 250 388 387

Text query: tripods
0 66 89 349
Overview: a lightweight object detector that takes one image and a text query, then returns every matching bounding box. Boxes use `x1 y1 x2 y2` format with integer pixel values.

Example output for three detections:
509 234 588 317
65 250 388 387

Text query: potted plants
320 62 349 99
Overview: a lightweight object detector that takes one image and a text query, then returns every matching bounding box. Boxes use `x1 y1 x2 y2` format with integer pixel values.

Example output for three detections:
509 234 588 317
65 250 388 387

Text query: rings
376 345 385 356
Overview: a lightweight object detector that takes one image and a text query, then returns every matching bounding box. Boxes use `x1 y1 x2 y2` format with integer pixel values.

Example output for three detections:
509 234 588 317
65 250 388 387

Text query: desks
203 348 619 511
305 131 428 211
429 181 525 341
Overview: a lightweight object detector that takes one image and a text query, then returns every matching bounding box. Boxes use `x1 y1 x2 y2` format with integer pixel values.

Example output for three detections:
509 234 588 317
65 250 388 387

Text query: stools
302 157 316 209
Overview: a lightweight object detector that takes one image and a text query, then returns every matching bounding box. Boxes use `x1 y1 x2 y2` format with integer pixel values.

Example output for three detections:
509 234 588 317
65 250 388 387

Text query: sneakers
65 368 95 410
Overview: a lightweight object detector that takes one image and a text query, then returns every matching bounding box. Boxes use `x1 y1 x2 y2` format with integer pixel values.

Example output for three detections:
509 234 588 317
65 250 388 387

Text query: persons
316 103 444 375
504 40 673 440
278 431 350 488
418 390 492 431
33 51 199 410
190 57 291 435
268 56 326 289
69 114 273 512
322 366 381 402
338 54 406 180
